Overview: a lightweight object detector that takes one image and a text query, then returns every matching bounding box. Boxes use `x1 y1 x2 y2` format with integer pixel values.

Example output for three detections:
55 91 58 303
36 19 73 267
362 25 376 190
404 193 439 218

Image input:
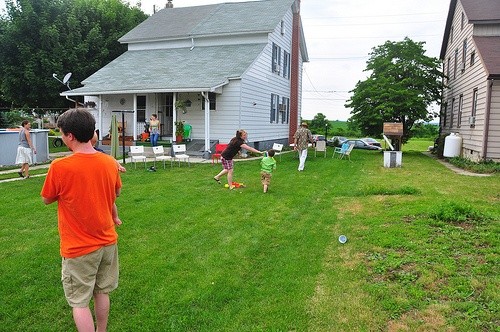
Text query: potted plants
174 121 185 142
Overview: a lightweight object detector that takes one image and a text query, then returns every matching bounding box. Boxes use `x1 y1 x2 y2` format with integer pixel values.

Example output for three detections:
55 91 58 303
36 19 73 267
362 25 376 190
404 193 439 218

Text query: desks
289 143 315 161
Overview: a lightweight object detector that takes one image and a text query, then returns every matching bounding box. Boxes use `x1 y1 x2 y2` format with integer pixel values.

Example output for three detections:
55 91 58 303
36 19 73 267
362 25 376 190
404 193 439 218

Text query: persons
213 129 264 190
260 150 276 193
293 122 314 171
148 113 160 146
40 109 125 332
16 120 36 179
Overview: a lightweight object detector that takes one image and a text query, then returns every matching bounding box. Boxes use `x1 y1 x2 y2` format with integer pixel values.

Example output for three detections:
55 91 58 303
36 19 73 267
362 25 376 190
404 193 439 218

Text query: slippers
229 185 235 189
214 177 221 184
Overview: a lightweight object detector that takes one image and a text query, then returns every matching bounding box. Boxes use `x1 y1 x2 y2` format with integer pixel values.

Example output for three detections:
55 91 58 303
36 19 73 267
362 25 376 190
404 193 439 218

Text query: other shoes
23 176 29 179
18 172 24 177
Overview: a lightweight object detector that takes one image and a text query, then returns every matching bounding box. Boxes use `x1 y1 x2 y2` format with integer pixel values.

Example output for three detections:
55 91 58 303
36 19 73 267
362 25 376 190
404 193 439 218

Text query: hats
300 122 308 126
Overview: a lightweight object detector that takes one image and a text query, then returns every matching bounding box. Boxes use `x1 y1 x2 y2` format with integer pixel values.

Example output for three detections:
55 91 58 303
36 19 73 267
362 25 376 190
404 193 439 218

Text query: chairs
130 146 146 169
273 143 283 162
117 121 127 137
315 141 327 158
332 143 355 160
172 144 190 168
212 143 228 164
153 145 172 169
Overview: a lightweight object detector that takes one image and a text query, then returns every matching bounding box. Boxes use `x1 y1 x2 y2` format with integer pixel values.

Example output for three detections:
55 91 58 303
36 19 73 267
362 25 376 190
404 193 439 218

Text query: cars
6 125 23 131
311 135 335 147
328 136 348 147
333 139 382 151
360 138 381 147
